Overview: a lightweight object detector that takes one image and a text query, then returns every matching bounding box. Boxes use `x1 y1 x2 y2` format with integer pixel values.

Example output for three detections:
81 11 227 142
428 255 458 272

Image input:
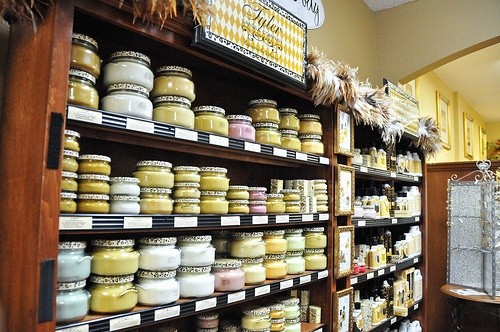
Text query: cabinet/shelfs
0 0 428 332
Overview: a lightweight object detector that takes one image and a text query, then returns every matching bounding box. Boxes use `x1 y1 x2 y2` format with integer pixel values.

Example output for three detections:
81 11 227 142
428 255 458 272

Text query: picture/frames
335 164 355 217
334 104 355 158
334 226 355 279
463 112 475 160
192 0 307 93
436 90 452 150
333 286 354 332
479 126 488 160
397 76 419 99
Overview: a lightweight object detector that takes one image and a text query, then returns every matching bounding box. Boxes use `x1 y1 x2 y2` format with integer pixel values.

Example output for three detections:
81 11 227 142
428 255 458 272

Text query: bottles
395 192 409 218
352 302 364 332
396 151 422 176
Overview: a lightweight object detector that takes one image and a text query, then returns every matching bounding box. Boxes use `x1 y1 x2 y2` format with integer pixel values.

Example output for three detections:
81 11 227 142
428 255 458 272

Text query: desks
440 284 500 332
